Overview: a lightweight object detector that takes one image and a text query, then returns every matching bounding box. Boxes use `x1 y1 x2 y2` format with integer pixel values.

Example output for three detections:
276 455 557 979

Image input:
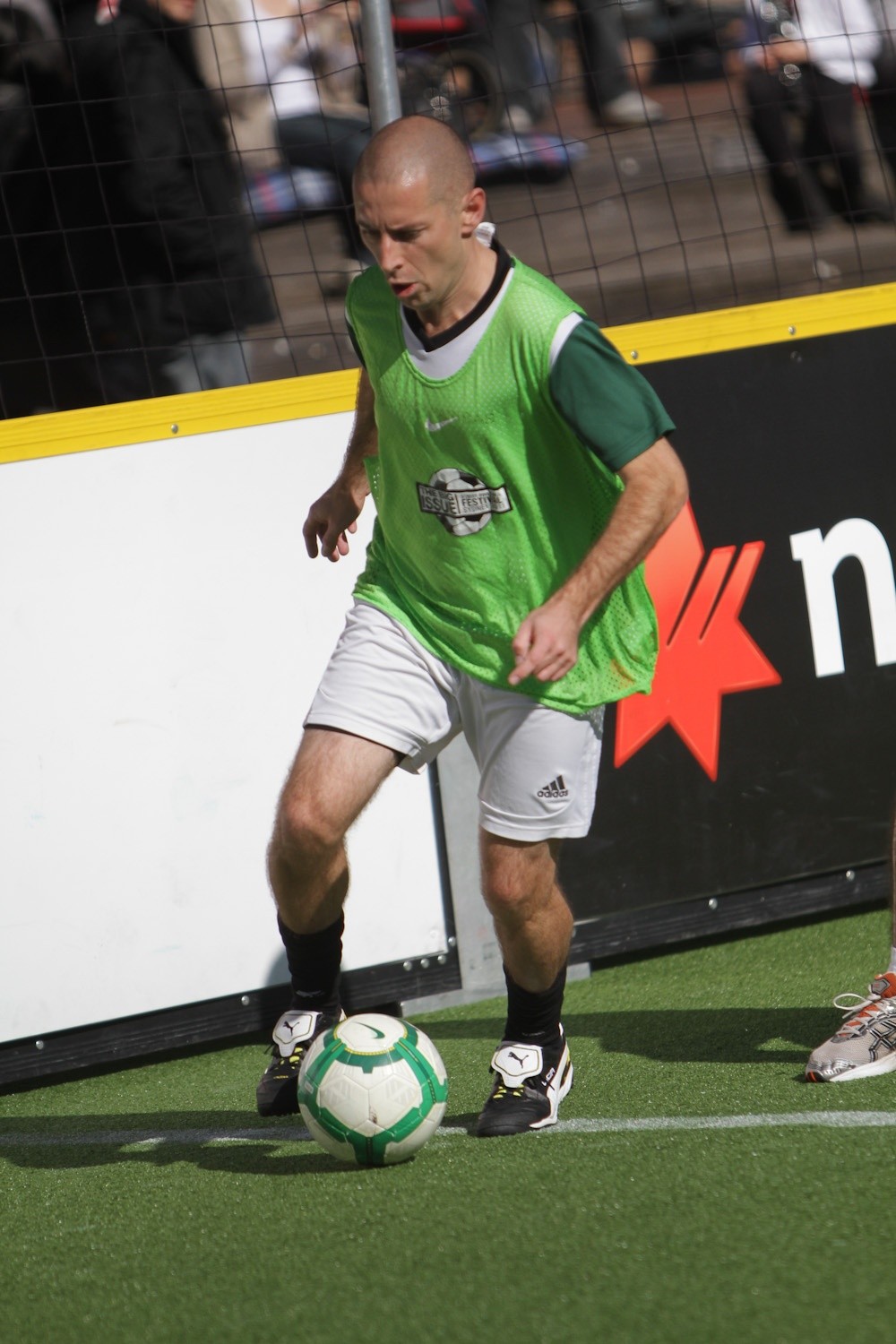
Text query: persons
0 0 896 421
256 115 689 1136
806 824 896 1082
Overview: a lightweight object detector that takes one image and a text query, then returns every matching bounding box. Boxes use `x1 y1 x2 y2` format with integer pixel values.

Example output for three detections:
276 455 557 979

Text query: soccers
296 1011 450 1168
420 466 496 540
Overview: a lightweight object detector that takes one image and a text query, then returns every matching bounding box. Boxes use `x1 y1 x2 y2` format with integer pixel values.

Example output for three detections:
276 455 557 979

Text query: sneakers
256 1005 346 1119
805 973 896 1083
475 1020 573 1138
